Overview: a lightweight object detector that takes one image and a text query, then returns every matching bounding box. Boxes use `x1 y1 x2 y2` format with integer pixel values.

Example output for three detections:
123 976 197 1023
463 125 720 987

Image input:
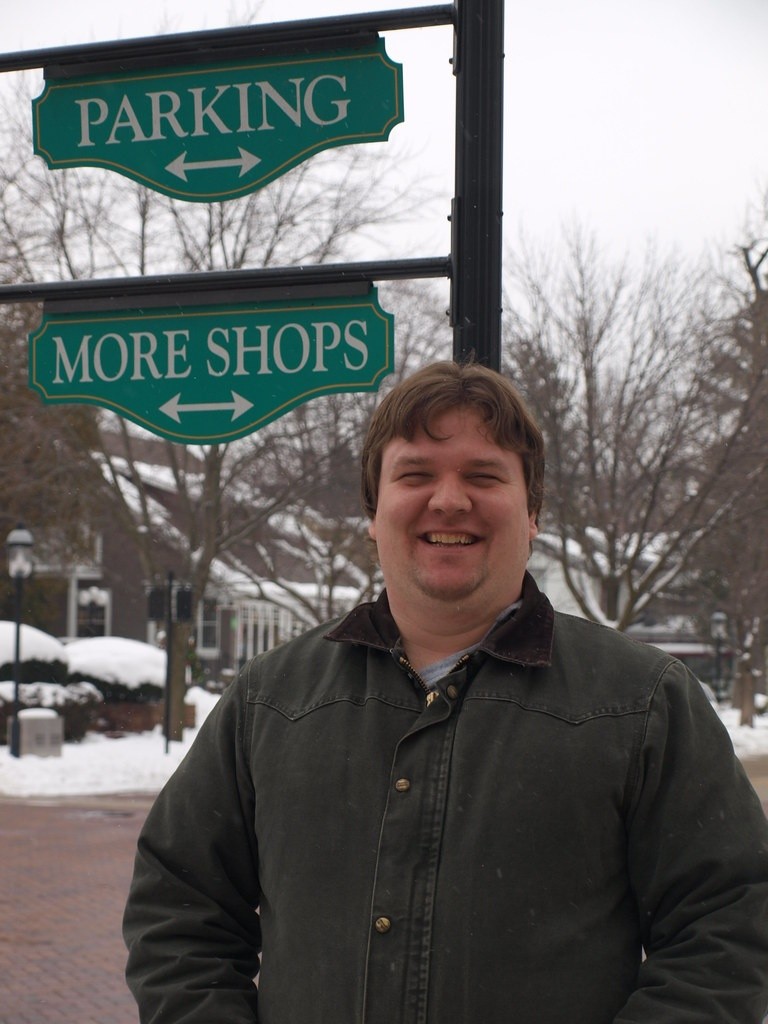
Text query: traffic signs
30 56 403 200
30 303 395 446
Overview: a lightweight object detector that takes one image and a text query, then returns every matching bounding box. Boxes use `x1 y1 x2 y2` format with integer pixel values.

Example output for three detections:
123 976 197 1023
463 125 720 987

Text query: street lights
5 520 34 756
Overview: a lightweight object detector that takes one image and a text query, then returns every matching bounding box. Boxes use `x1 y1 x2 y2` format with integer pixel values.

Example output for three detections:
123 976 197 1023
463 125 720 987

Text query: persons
123 364 768 1023
